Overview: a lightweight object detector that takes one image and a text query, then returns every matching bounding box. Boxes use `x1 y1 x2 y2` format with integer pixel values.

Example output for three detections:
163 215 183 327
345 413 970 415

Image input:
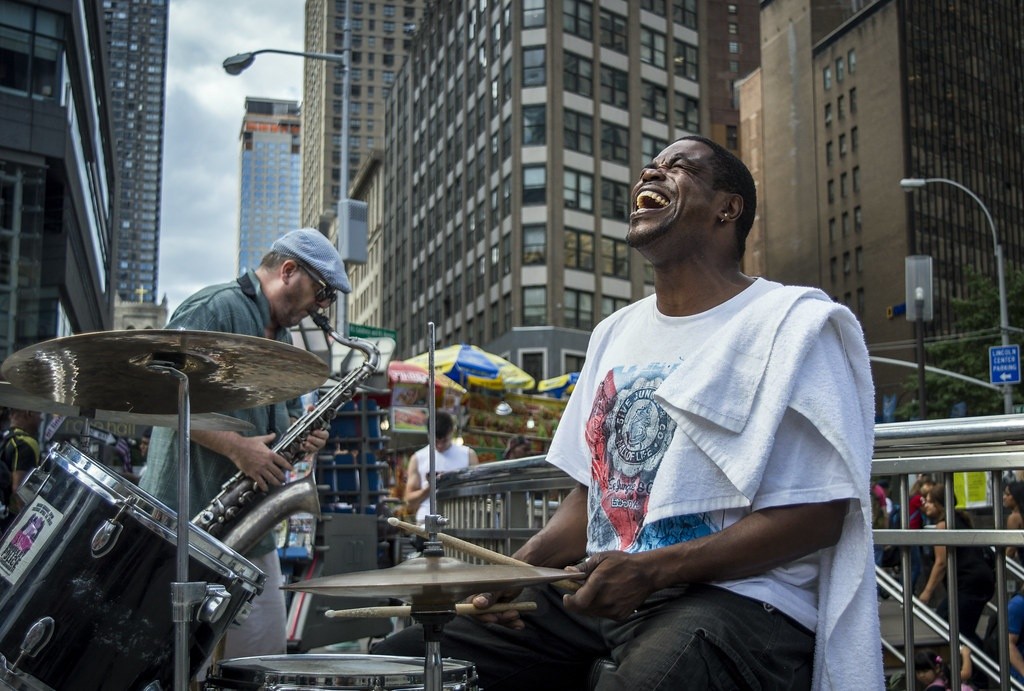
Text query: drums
2 438 269 691
203 648 480 691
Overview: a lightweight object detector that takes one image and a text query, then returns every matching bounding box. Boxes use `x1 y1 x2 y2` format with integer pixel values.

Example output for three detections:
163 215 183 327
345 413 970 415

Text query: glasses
297 262 337 306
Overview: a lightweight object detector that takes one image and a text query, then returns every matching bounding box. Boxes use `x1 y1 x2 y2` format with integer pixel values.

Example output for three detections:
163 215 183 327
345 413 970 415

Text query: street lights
898 177 1014 417
222 0 371 338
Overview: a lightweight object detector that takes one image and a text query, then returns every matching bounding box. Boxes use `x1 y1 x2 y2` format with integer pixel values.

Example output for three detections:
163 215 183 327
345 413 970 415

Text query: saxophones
188 308 386 555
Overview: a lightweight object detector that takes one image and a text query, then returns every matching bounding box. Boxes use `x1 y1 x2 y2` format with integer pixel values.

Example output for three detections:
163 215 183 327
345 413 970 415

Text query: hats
271 227 352 294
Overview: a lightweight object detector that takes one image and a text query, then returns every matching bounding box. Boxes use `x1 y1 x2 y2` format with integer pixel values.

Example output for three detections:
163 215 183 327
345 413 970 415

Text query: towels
643 284 887 691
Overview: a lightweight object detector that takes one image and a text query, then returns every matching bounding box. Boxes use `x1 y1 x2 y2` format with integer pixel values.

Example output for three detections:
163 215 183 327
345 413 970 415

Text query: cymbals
275 552 589 597
1 328 331 418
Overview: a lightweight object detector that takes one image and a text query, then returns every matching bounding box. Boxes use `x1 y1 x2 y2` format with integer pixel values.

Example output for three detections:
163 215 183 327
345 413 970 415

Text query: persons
865 470 1024 691
129 228 353 681
369 136 861 691
121 427 152 486
403 410 479 550
0 409 44 521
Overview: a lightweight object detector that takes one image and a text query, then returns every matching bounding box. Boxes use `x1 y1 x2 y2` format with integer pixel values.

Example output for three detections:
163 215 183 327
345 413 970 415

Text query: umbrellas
387 341 582 440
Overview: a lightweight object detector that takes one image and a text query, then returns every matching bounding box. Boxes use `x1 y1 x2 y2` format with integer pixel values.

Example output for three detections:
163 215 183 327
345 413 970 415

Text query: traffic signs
987 344 1023 386
